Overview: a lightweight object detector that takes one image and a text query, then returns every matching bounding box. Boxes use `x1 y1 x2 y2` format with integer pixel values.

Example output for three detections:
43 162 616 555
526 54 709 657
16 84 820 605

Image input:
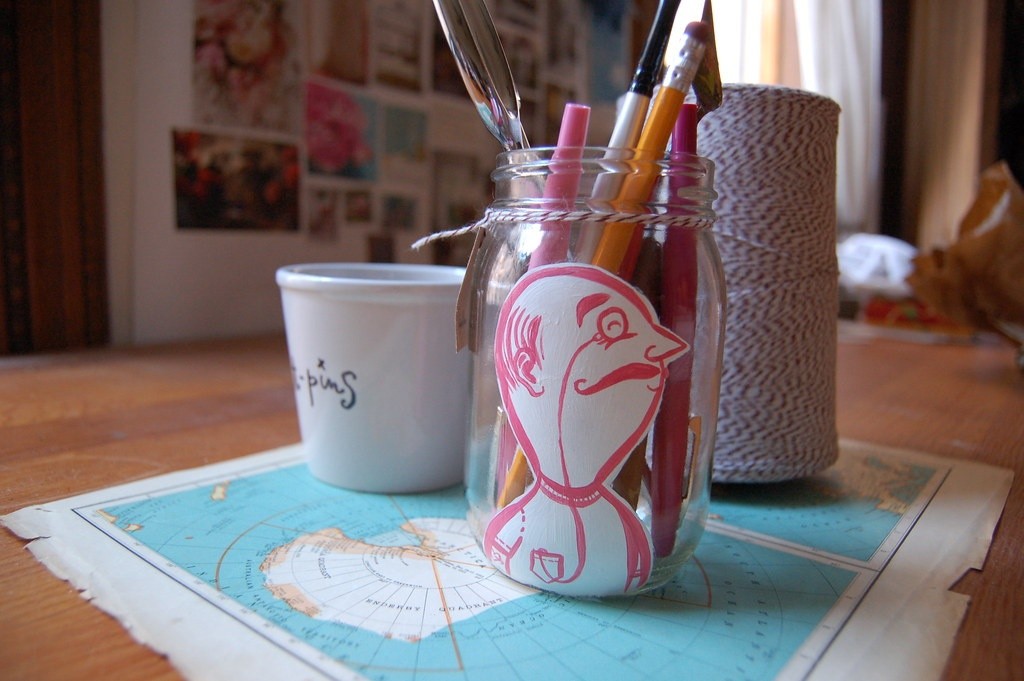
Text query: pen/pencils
498 0 715 558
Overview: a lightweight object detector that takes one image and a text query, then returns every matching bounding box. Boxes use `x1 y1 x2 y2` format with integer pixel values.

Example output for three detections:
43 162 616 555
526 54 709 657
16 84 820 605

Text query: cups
276 263 471 493
470 145 728 597
